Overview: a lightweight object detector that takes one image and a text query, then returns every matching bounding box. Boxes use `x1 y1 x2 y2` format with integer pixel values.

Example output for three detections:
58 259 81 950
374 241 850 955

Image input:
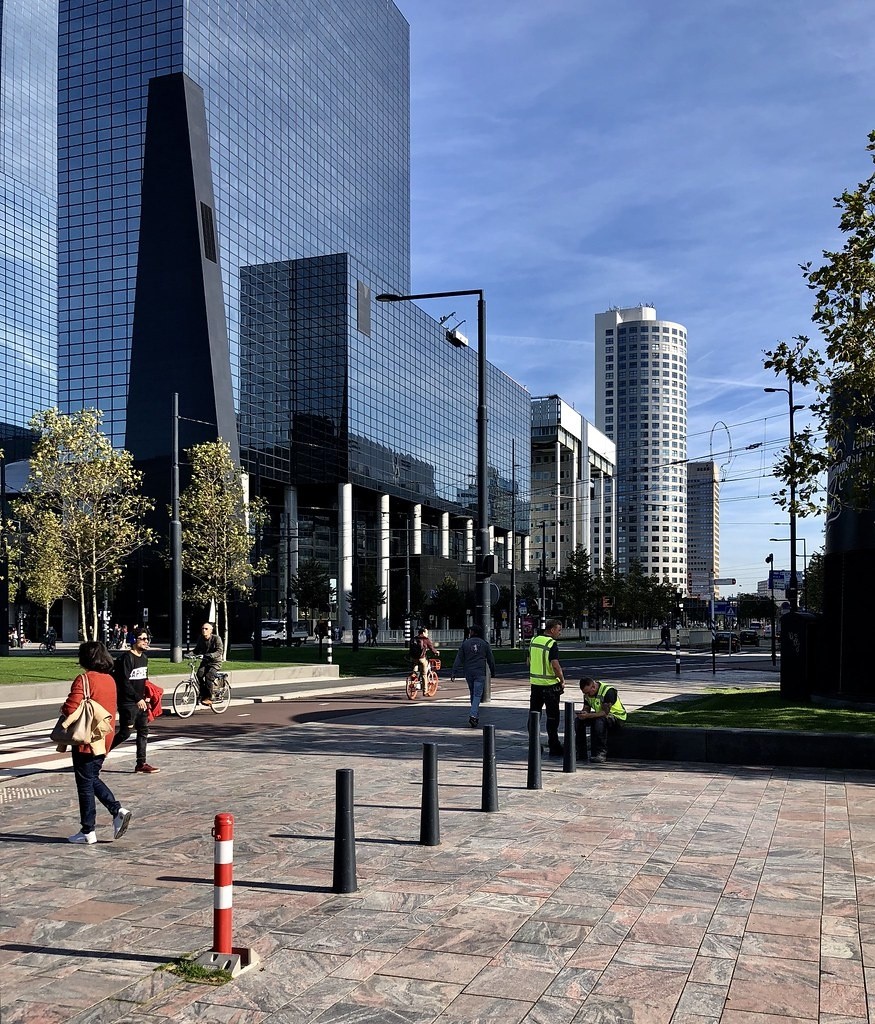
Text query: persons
363 624 378 647
463 626 469 643
60 641 132 844
109 629 160 773
9 627 17 640
657 626 671 650
187 623 223 705
146 626 152 645
331 624 345 640
108 624 139 650
410 628 440 696
451 626 495 727
575 677 627 763
47 626 55 652
314 624 318 641
496 627 503 647
527 621 565 754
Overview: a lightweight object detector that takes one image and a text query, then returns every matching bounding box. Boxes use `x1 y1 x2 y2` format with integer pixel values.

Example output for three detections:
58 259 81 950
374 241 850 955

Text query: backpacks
409 639 424 658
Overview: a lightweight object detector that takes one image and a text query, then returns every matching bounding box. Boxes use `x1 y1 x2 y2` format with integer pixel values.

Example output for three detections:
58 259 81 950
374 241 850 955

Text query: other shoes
469 717 479 727
202 699 213 705
590 754 607 762
423 691 429 696
549 746 564 753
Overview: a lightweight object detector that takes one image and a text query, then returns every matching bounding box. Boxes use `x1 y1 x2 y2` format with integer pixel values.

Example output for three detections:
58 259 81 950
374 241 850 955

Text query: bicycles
39 633 56 656
172 654 233 718
405 653 441 700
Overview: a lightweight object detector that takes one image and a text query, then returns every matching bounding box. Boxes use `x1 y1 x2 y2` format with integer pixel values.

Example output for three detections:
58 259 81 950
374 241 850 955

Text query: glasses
137 637 149 640
202 628 209 630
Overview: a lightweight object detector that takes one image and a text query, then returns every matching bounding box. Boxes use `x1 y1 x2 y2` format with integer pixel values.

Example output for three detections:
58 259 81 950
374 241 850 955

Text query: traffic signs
714 578 736 585
689 580 710 586
697 593 711 600
688 572 710 578
688 586 710 594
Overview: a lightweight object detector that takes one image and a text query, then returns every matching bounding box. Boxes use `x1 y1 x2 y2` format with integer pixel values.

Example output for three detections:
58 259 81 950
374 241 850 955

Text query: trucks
250 620 309 648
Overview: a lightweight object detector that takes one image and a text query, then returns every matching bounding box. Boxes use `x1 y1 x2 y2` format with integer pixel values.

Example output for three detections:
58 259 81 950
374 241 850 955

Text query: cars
750 621 780 640
740 630 760 647
711 632 741 653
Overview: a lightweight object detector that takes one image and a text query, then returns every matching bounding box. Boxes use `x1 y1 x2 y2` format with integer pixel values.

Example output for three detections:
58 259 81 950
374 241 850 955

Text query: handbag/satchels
49 673 94 745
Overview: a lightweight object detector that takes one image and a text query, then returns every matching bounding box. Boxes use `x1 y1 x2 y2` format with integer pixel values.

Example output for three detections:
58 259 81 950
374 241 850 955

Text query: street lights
762 378 804 610
769 537 809 613
375 287 494 704
764 553 778 666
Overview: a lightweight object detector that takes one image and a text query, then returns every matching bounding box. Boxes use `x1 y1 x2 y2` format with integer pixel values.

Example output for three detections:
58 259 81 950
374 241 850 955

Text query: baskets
429 659 441 670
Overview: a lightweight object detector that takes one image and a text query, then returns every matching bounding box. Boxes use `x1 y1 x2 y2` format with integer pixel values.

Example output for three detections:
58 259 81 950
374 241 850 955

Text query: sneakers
68 830 97 843
112 808 132 839
135 763 161 773
